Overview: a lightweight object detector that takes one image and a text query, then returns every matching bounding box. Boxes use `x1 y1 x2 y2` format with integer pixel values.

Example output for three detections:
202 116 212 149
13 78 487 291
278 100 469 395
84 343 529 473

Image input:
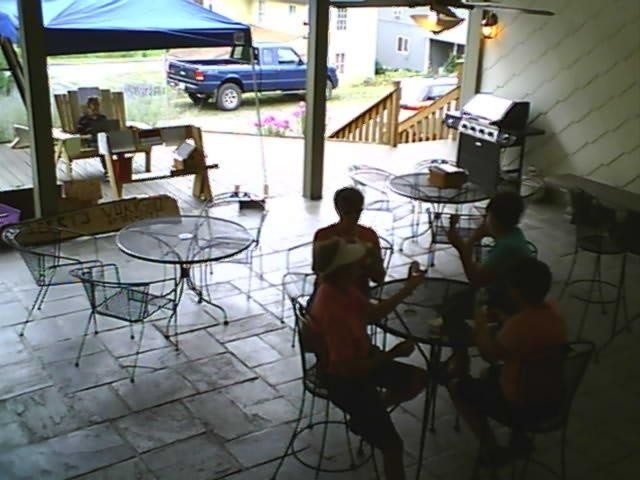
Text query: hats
315 237 366 276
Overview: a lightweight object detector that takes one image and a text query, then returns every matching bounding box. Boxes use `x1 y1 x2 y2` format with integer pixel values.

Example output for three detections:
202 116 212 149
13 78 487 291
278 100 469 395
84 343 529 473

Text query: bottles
475 286 489 314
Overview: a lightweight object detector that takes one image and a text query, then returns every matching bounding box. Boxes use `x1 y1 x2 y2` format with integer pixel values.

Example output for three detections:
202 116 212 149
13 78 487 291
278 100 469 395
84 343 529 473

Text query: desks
115 215 256 355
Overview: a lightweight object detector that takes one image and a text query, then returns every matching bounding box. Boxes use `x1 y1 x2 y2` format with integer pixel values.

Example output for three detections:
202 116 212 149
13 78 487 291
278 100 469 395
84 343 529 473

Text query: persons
442 193 532 374
447 256 570 468
77 97 111 182
309 238 429 480
312 187 385 308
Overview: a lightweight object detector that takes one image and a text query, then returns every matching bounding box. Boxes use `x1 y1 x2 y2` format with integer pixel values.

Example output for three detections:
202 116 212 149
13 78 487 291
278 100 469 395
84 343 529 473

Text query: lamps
409 5 467 36
481 10 498 39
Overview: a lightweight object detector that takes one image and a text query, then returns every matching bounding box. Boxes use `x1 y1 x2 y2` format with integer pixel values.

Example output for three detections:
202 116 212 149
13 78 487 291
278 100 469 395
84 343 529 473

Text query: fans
329 0 558 20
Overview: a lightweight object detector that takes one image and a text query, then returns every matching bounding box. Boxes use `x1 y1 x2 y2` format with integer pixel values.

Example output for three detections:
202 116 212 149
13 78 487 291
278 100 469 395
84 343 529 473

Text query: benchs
50 119 220 210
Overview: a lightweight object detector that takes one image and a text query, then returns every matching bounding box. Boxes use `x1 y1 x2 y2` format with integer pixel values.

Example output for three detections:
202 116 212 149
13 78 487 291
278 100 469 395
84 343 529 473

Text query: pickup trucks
166 43 338 111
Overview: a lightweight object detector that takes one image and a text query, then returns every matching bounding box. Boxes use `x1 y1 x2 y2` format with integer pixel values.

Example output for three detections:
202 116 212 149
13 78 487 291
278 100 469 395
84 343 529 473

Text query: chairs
66 260 182 386
183 189 267 303
1 220 109 336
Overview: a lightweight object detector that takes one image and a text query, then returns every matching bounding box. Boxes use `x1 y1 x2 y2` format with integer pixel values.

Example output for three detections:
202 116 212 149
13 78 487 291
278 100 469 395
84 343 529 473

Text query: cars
397 76 460 120
